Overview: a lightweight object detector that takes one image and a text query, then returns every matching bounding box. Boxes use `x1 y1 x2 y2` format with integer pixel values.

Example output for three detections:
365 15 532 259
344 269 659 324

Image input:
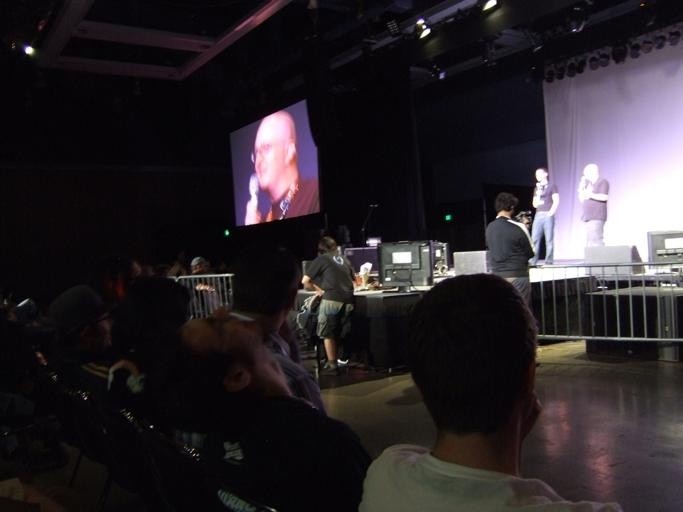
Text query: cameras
515 211 533 225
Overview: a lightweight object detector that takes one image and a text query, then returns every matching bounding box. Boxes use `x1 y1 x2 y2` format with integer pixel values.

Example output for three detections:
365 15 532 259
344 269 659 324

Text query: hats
190 256 210 269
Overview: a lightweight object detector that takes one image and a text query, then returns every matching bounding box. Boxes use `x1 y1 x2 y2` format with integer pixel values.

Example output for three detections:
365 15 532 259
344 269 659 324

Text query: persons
529 168 559 269
358 274 623 511
485 192 542 367
243 111 320 226
577 163 609 245
1 234 363 511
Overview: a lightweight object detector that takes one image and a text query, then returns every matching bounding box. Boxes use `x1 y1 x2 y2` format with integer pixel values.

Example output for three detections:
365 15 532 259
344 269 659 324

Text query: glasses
250 140 277 163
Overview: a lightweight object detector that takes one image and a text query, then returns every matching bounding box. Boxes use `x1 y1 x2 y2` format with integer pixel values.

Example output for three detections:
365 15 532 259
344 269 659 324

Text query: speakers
453 251 493 275
583 245 646 274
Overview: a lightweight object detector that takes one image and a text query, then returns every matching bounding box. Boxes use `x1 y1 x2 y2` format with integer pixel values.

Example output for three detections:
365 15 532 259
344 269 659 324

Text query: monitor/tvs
648 231 683 265
378 240 433 293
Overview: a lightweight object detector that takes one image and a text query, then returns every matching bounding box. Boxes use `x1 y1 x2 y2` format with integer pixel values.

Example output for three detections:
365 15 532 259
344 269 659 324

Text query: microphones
577 176 586 193
244 174 259 225
536 183 540 190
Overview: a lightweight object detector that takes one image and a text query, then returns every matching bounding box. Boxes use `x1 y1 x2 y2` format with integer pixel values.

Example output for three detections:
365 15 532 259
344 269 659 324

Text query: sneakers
316 356 350 376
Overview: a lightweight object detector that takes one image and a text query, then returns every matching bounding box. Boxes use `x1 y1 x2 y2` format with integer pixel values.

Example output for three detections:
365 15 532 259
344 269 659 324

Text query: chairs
69 384 278 511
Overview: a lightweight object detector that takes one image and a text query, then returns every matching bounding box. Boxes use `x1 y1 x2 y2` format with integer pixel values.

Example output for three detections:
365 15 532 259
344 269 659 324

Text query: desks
297 286 423 372
